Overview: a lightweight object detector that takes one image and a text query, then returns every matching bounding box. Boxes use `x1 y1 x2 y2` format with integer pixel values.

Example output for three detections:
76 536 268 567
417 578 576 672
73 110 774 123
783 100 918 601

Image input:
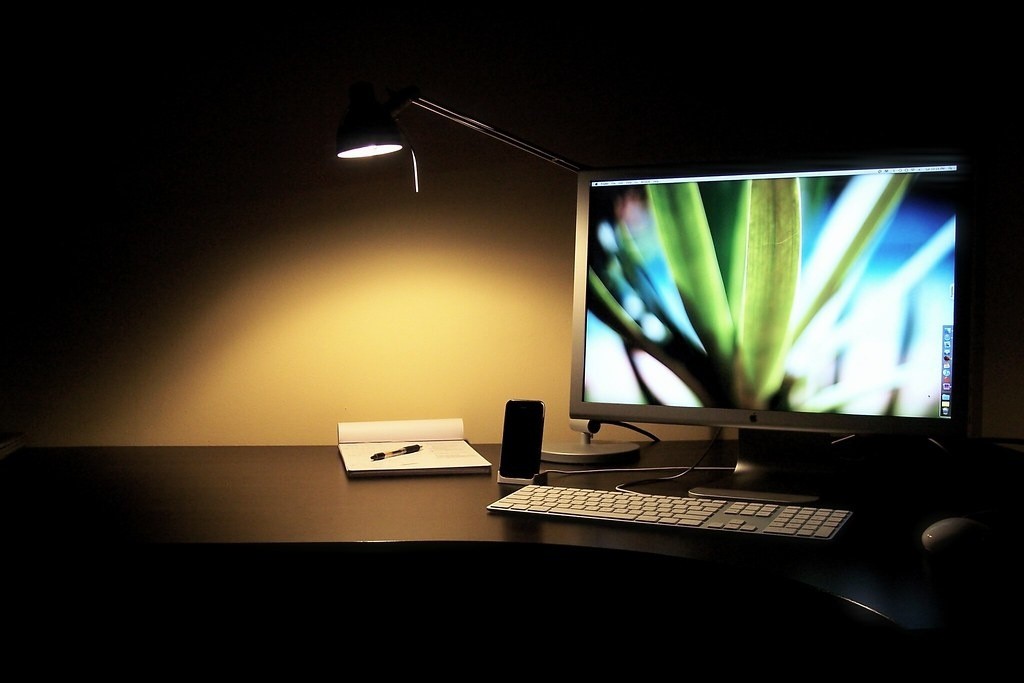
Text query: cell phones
500 399 545 479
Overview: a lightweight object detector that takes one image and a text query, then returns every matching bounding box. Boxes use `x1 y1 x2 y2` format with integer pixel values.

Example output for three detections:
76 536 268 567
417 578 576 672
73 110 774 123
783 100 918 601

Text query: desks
0 434 1024 683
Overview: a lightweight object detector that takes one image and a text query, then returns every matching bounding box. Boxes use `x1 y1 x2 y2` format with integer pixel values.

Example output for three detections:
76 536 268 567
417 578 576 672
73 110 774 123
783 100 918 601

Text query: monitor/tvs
569 157 982 505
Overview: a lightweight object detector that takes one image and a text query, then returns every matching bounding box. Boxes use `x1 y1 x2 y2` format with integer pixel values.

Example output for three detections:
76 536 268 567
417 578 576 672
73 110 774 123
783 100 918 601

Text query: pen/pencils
370 444 422 460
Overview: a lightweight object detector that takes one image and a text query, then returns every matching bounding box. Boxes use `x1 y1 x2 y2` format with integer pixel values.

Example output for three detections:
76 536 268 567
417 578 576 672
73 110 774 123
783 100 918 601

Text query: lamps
335 78 587 178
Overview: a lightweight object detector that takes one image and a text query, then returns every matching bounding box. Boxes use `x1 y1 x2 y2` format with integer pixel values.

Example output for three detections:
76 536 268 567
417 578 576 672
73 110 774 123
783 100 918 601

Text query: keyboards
485 485 856 542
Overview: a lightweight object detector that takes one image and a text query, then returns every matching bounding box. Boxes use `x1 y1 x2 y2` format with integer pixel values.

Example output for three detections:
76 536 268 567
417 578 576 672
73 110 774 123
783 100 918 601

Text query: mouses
922 518 980 552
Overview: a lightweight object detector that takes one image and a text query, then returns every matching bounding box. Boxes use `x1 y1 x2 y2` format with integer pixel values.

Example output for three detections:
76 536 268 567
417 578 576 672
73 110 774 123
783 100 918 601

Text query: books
336 420 493 480
